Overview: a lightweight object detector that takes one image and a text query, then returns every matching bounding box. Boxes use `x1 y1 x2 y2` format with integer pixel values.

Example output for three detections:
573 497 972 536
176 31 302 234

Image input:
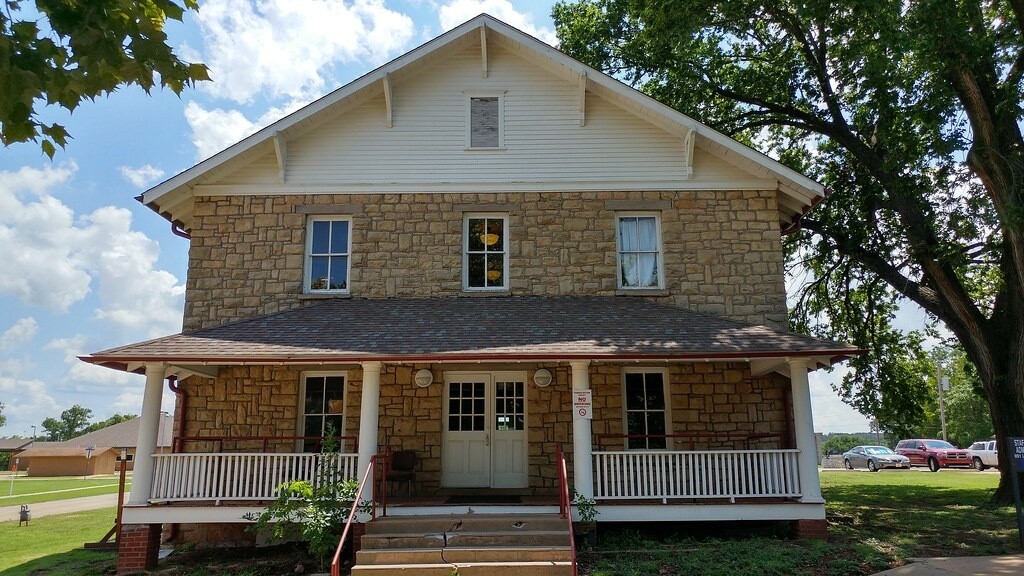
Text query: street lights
56 431 59 441
15 459 19 476
160 411 170 454
31 425 37 448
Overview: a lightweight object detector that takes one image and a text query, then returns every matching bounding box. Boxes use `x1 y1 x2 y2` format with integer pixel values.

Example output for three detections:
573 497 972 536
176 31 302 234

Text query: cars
842 446 911 471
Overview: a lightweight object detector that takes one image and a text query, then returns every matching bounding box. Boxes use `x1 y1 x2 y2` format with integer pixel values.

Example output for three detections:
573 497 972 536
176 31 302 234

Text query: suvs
895 438 973 473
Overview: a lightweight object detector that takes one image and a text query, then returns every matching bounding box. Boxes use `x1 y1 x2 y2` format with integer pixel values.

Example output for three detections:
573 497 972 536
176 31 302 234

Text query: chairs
376 450 419 501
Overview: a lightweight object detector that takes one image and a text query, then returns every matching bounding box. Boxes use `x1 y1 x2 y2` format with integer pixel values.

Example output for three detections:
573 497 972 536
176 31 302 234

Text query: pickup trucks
966 440 998 471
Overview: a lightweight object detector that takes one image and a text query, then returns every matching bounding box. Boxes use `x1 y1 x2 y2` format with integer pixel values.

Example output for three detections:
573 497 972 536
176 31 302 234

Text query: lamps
415 370 433 387
486 262 501 281
534 369 551 388
478 227 499 246
